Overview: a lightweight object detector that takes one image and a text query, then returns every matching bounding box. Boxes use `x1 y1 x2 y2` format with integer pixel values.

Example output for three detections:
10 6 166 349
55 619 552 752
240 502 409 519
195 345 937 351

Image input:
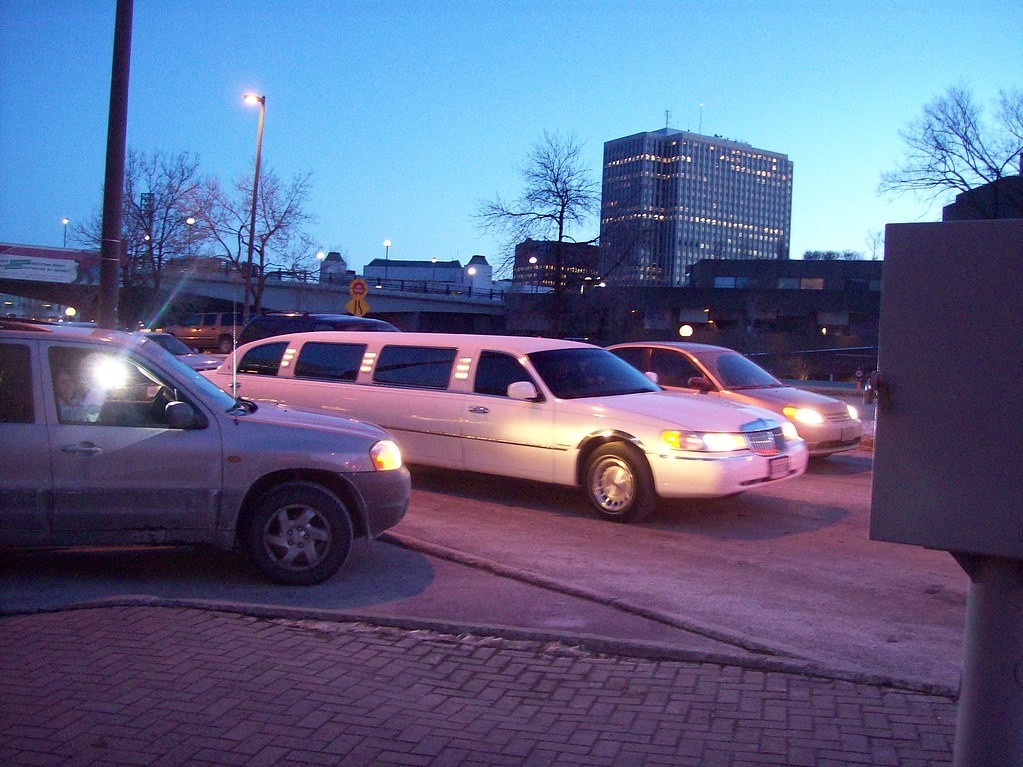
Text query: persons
567 353 605 389
52 367 89 426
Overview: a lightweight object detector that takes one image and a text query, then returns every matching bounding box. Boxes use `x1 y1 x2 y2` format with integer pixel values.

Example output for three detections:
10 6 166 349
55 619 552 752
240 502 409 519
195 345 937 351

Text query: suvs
158 311 246 353
234 310 402 352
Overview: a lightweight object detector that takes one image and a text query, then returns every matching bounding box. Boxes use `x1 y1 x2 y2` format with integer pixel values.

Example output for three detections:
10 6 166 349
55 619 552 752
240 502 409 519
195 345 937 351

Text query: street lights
529 257 537 292
468 267 475 289
431 256 437 288
678 325 693 343
63 217 70 247
383 240 391 283
187 217 195 255
65 307 76 322
317 252 325 277
243 92 265 321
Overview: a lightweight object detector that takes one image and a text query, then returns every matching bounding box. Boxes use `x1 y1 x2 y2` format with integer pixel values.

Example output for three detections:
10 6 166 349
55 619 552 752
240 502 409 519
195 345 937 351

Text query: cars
863 370 877 404
0 328 410 584
133 332 224 374
579 341 862 459
195 331 809 522
